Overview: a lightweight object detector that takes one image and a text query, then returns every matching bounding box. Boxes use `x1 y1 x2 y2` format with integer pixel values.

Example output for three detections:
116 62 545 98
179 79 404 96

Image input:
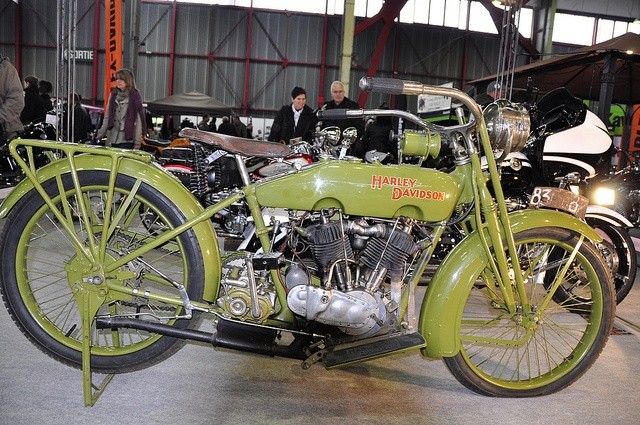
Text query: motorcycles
590 145 640 227
0 77 617 407
239 86 636 316
140 125 359 242
141 137 194 156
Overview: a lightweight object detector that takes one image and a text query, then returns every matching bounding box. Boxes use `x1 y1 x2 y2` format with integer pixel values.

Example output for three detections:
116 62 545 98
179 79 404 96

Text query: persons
97 70 142 150
62 92 92 141
38 81 53 122
231 115 251 137
468 80 507 125
211 118 217 130
314 81 365 156
270 86 319 145
199 114 210 131
20 77 41 122
219 116 236 130
0 55 24 137
